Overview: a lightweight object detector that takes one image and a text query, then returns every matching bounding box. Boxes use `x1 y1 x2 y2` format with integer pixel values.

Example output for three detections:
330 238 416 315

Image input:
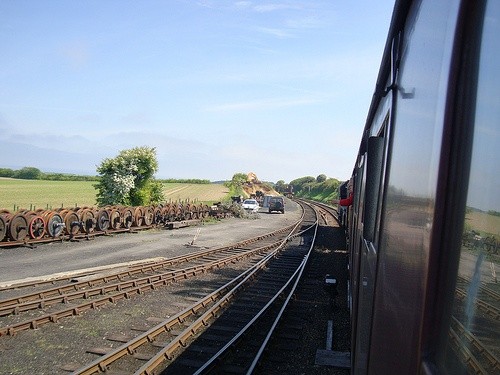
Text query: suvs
269 197 284 214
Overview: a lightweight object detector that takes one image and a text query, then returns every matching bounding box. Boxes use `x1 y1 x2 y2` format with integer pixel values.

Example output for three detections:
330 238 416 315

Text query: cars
242 199 259 213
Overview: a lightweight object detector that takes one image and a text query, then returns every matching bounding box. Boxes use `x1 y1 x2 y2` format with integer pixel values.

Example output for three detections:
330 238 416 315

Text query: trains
338 0 500 375
